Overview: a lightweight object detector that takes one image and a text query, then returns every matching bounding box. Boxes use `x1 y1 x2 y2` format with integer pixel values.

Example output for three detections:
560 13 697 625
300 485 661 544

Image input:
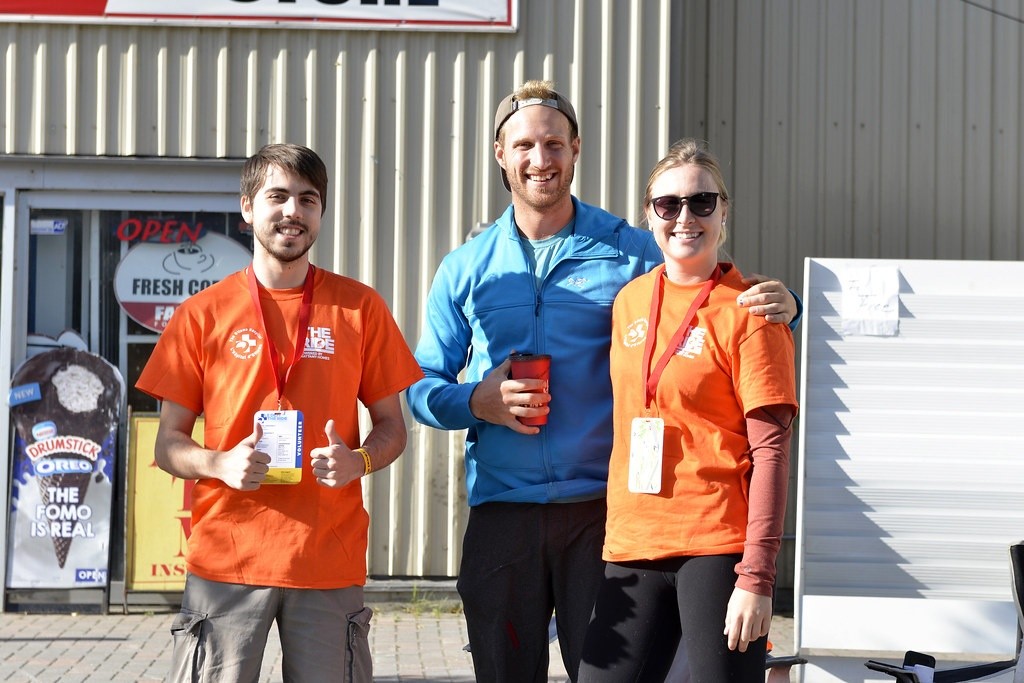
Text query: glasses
647 192 722 220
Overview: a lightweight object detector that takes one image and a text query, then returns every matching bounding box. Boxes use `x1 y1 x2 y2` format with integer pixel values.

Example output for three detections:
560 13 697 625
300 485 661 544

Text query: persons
134 145 427 683
404 79 802 683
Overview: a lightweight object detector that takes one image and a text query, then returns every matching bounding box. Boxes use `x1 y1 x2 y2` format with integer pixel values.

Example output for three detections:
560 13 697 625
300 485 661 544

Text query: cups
509 352 551 425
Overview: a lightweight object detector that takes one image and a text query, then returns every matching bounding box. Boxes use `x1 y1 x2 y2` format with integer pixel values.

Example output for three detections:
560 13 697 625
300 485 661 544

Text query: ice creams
10 347 120 567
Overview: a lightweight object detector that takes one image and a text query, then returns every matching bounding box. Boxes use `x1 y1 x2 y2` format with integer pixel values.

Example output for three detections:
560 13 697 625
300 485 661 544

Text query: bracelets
352 448 371 476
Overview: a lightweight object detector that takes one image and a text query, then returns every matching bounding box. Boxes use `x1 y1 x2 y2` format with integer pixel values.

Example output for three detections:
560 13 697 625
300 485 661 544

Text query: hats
494 88 578 192
